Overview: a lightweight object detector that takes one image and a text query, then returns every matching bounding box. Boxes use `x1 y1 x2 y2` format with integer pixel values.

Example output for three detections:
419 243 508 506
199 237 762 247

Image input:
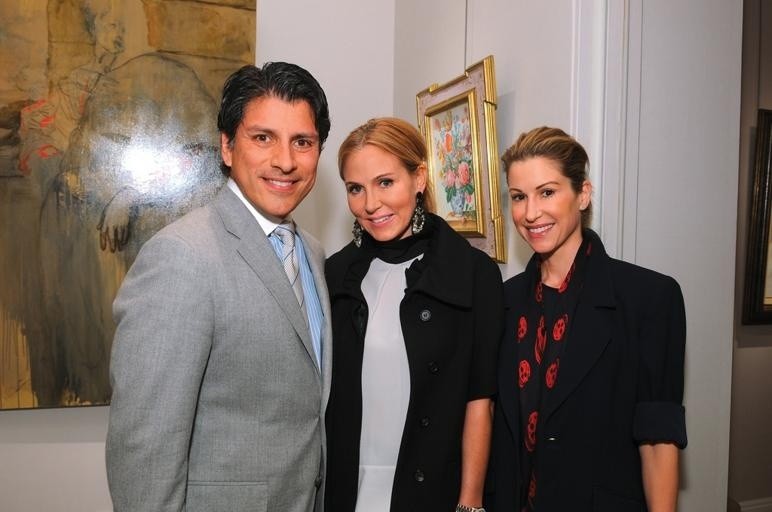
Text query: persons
321 116 504 512
103 58 337 511
482 126 688 512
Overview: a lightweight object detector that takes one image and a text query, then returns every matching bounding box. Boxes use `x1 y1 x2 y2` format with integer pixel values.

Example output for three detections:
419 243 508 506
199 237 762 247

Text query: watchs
454 504 486 512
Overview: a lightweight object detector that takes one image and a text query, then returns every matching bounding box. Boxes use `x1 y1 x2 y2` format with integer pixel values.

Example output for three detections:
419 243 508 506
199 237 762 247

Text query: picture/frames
740 103 771 333
407 53 507 266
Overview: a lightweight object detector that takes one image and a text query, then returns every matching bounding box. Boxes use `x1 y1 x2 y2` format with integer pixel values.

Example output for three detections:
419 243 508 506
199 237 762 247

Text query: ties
273 221 311 338
